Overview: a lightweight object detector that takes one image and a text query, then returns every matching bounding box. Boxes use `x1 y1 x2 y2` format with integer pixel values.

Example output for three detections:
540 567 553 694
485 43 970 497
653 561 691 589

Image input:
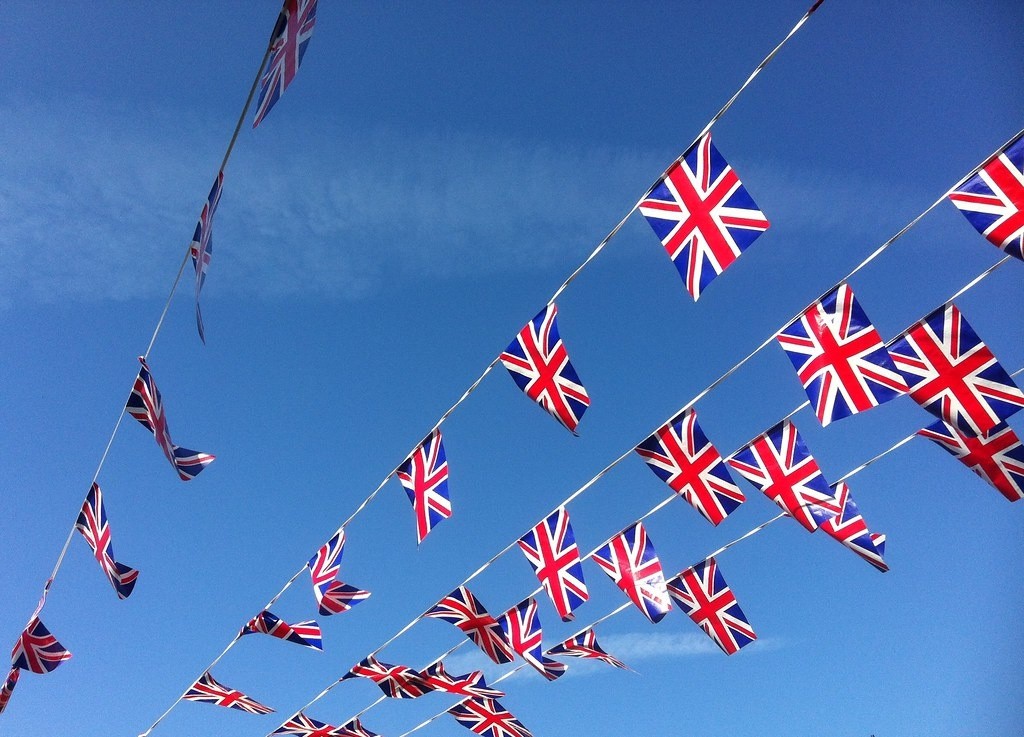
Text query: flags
0 1 1024 737
